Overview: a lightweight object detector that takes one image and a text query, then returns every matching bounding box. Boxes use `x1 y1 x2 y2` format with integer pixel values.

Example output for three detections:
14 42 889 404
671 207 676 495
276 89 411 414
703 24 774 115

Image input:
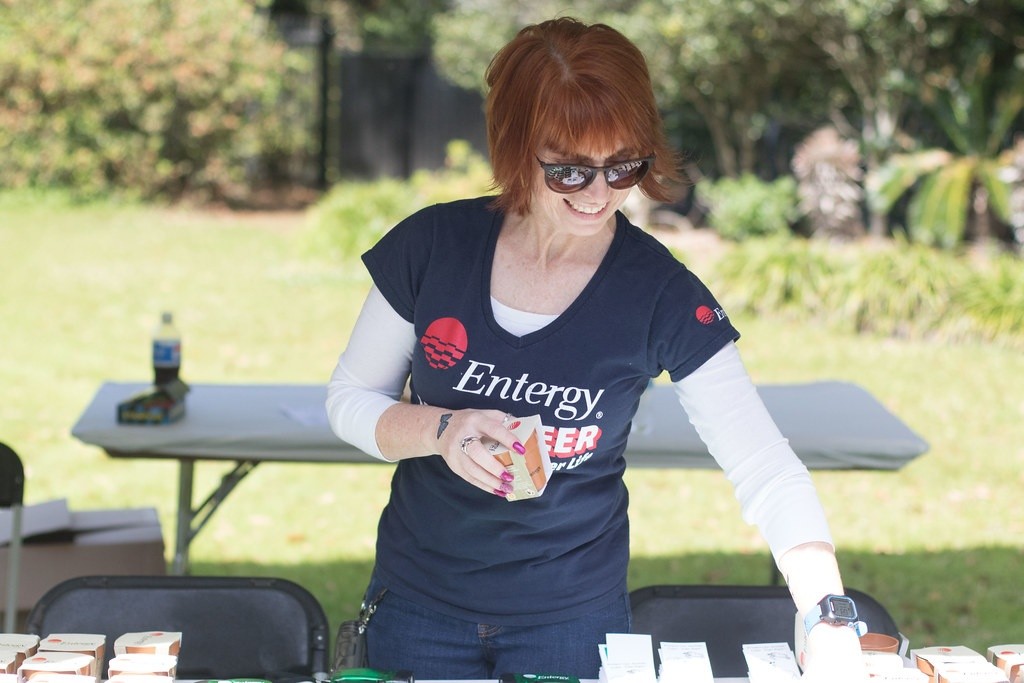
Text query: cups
493 427 546 491
0 639 179 683
858 632 1024 683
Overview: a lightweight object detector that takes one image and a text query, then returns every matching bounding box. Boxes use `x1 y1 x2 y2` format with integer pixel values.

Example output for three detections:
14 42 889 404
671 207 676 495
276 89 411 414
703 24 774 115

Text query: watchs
803 594 868 638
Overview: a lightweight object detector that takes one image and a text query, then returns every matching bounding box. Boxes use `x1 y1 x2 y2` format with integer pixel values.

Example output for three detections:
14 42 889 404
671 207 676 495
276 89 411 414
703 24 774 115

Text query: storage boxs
0 499 165 611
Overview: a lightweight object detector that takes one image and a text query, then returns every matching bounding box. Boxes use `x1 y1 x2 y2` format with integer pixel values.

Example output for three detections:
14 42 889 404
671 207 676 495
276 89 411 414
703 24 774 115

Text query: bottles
153 312 179 387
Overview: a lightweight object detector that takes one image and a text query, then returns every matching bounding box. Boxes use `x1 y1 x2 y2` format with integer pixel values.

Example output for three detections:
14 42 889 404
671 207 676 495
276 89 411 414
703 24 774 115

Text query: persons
325 13 865 683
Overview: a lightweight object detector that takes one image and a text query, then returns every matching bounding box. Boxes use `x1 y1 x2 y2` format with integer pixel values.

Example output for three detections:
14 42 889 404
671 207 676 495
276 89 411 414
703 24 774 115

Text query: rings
461 436 481 455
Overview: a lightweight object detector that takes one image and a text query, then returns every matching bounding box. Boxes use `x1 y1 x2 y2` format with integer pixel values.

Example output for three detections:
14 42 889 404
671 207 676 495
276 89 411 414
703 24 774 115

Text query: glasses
532 148 659 195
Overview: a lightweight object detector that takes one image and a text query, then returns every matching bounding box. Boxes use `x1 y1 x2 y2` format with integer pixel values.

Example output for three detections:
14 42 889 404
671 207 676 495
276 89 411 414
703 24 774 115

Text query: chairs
0 443 25 634
629 585 909 679
23 575 330 680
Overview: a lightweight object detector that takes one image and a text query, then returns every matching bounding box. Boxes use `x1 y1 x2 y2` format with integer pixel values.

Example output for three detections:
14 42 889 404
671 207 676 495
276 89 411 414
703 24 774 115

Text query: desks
73 382 931 589
